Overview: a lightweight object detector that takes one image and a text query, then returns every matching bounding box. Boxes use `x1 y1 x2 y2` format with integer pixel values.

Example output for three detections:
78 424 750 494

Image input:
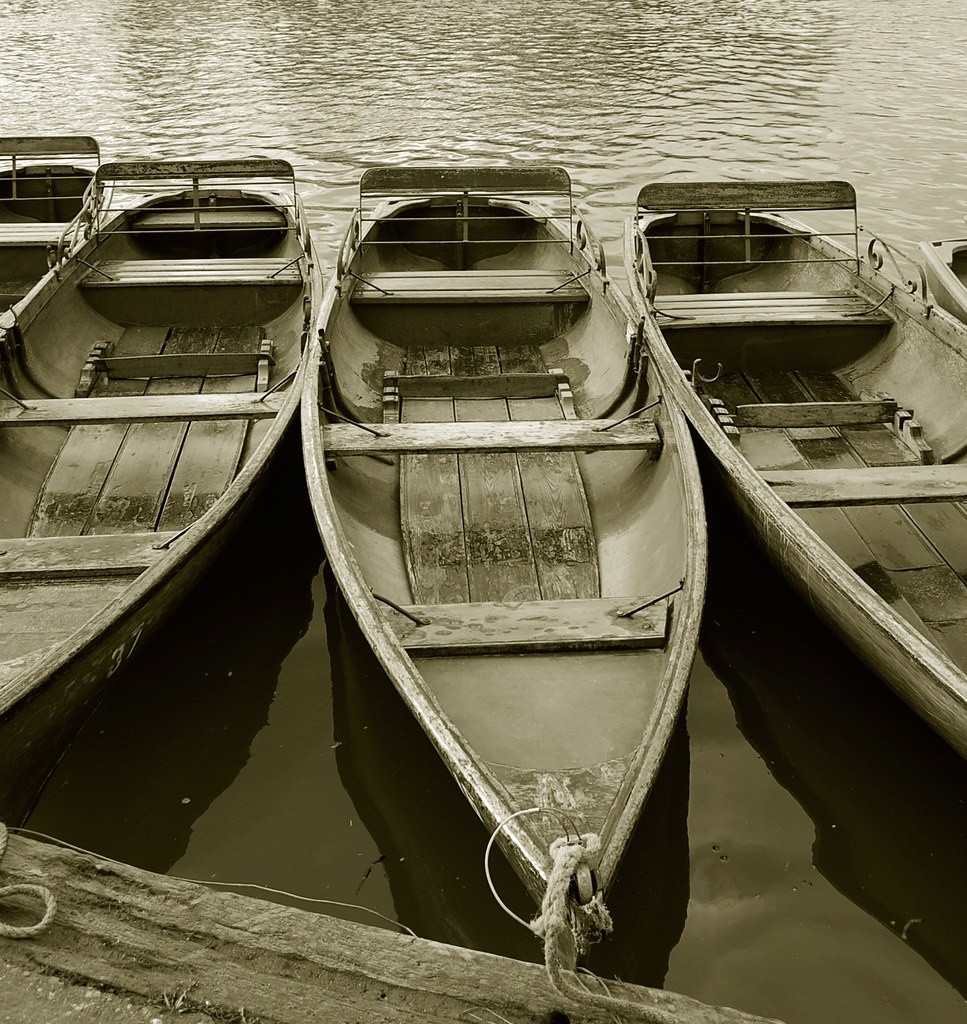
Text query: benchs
322 417 665 469
654 287 896 334
754 464 967 507
0 221 87 246
346 268 589 309
77 257 303 288
0 372 297 428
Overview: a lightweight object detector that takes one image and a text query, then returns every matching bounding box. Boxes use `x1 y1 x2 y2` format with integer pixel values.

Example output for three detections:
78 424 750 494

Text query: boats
624 181 967 760
0 159 321 790
0 136 115 318
919 238 967 326
303 163 709 957
